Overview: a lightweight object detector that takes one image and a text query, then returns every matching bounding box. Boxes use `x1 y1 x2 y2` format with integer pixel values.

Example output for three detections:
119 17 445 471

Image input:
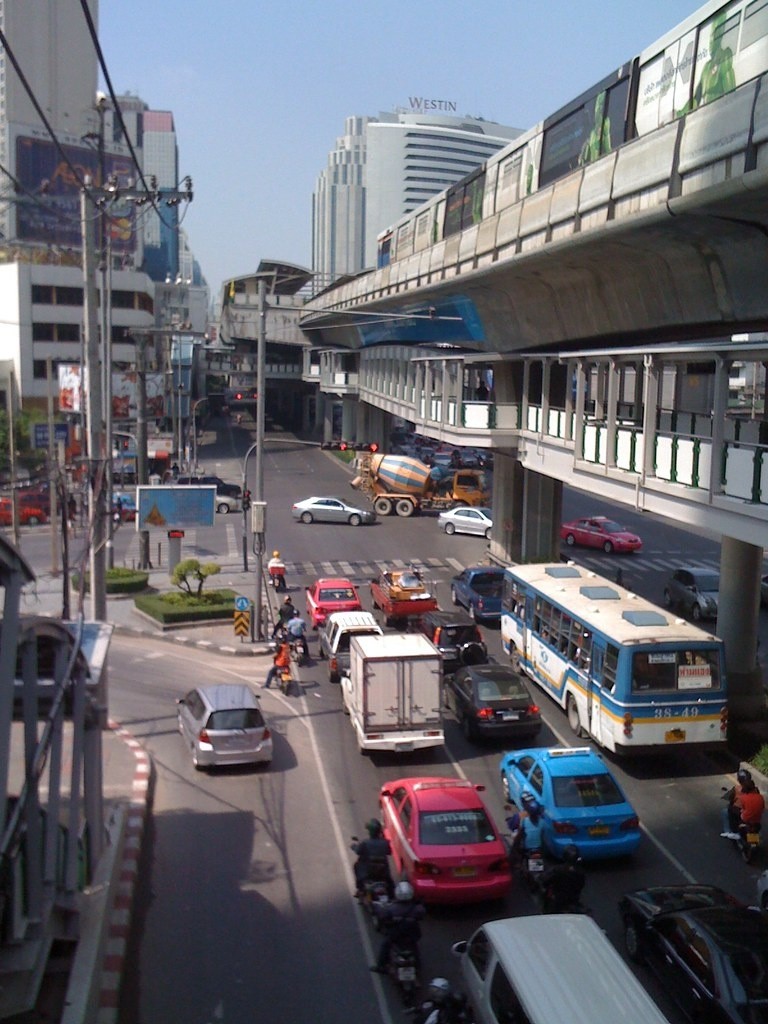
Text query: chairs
483 689 490 694
509 686 518 694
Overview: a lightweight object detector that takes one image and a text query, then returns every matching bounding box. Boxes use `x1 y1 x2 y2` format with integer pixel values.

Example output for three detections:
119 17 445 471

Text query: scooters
268 645 293 696
536 858 594 918
262 562 284 593
282 623 306 667
365 891 426 1008
504 802 544 894
351 835 390 930
721 785 761 865
274 606 290 644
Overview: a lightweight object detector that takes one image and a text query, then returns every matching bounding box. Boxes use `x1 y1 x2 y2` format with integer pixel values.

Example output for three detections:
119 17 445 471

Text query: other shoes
260 684 269 689
271 634 276 639
728 833 741 839
284 586 289 590
353 891 366 898
720 832 730 837
370 965 389 975
305 655 313 663
267 579 273 585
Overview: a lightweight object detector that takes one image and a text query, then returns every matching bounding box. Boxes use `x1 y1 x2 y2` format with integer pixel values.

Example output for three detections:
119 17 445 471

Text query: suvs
402 610 488 674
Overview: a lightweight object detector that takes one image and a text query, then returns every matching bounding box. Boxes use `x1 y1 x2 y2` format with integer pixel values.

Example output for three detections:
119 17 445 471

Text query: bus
500 560 731 758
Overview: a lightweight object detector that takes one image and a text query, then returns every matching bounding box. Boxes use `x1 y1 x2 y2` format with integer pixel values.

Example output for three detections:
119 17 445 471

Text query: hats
429 977 448 989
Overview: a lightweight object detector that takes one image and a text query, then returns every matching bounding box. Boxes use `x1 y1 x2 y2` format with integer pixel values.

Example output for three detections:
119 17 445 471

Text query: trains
376 0 768 267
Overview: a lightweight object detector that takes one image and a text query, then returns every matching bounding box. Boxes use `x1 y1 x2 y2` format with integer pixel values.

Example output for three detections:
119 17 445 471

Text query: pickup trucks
368 569 439 627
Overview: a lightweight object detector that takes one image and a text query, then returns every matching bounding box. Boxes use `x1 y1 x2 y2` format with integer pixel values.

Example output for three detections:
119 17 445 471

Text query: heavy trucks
350 452 492 516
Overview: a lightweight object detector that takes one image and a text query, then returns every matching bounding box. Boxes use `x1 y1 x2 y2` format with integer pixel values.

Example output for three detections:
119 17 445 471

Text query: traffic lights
229 387 258 399
353 441 381 453
242 490 252 509
320 440 348 452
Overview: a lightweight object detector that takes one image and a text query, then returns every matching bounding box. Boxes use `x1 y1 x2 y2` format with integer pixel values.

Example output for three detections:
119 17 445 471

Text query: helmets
564 845 579 863
285 595 292 603
521 792 535 806
280 632 289 639
395 882 414 900
528 800 541 815
273 550 280 558
293 609 300 616
738 770 751 785
365 818 381 832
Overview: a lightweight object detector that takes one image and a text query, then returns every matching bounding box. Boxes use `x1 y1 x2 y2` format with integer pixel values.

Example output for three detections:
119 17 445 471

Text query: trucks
340 634 446 755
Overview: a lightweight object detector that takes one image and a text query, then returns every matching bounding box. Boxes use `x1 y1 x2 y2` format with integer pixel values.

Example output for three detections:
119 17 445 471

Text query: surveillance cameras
97 93 105 102
430 307 436 315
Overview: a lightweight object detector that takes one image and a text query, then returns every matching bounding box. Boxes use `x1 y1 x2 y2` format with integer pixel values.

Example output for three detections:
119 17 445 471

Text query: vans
443 663 542 743
449 912 672 1024
316 609 386 684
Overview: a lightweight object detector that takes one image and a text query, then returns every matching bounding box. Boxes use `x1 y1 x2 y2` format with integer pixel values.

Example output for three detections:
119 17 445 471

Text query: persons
720 769 765 841
285 608 309 657
349 818 393 898
507 792 546 865
424 453 435 464
269 595 295 639
419 976 466 1024
368 881 425 975
541 844 578 888
267 550 286 590
261 635 291 688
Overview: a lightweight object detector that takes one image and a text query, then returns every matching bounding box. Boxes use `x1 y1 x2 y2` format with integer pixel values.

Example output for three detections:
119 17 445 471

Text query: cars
174 682 274 770
618 883 768 1024
177 474 241 497
561 516 643 555
16 488 61 515
498 746 644 867
761 574 768 607
662 566 720 622
0 497 48 527
397 419 491 470
291 496 377 526
450 564 507 622
436 505 493 540
216 494 238 513
305 576 361 632
111 492 135 521
376 777 513 907
